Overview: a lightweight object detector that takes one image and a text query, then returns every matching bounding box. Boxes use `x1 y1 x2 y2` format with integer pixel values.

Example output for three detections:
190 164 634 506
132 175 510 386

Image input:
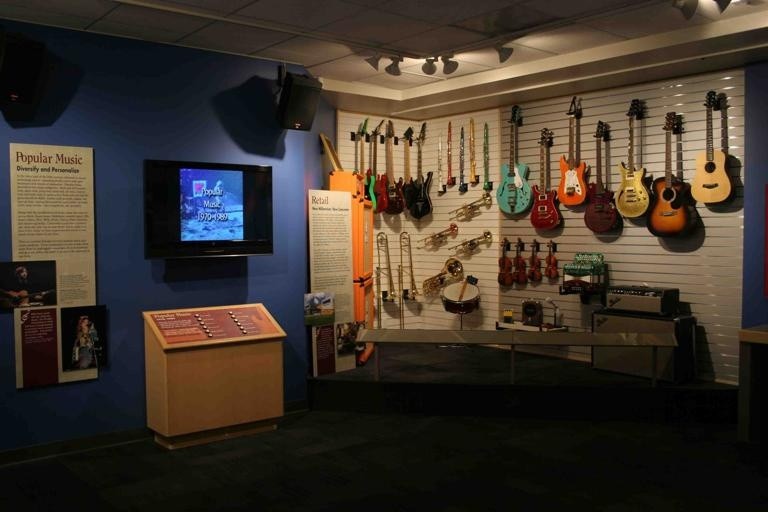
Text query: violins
512 237 528 283
545 240 558 278
498 239 513 286
527 239 542 281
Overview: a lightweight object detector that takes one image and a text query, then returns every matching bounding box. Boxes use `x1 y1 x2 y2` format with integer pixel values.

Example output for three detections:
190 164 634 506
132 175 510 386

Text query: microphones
546 296 559 311
85 322 92 327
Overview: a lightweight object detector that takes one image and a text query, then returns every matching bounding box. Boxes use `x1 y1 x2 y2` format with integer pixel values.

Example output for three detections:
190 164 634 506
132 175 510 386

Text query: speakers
0 37 82 128
522 300 541 326
275 71 322 133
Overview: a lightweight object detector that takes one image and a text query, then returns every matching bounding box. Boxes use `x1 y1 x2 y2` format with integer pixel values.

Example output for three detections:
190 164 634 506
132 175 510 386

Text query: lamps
363 45 458 76
671 1 732 20
492 42 514 63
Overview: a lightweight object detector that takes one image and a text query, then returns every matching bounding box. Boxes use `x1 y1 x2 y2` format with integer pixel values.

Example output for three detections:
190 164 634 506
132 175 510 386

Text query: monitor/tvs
143 158 274 260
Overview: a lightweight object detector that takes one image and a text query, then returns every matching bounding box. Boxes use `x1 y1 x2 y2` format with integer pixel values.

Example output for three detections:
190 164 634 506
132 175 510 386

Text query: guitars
497 105 534 215
646 112 692 236
410 123 433 218
614 98 654 219
584 120 618 234
403 127 414 209
385 120 406 215
362 134 377 212
691 91 735 206
556 96 588 207
371 129 389 213
531 128 562 230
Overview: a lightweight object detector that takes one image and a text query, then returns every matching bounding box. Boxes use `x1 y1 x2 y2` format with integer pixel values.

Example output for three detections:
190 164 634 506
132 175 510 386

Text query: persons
12 266 30 302
74 316 97 370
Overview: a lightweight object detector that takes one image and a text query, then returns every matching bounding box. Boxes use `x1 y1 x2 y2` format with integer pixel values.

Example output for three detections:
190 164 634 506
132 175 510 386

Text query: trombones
399 232 422 328
376 231 400 329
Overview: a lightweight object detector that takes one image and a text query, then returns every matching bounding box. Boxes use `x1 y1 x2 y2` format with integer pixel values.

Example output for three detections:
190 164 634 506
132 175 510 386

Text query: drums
439 281 481 314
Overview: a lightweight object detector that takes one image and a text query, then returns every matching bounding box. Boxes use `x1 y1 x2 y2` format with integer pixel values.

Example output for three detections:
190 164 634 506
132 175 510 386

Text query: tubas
423 259 463 297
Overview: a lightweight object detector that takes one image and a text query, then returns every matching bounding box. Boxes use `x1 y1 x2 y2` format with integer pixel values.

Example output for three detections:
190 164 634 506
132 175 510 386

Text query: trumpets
447 229 493 255
415 223 458 249
447 193 492 221
